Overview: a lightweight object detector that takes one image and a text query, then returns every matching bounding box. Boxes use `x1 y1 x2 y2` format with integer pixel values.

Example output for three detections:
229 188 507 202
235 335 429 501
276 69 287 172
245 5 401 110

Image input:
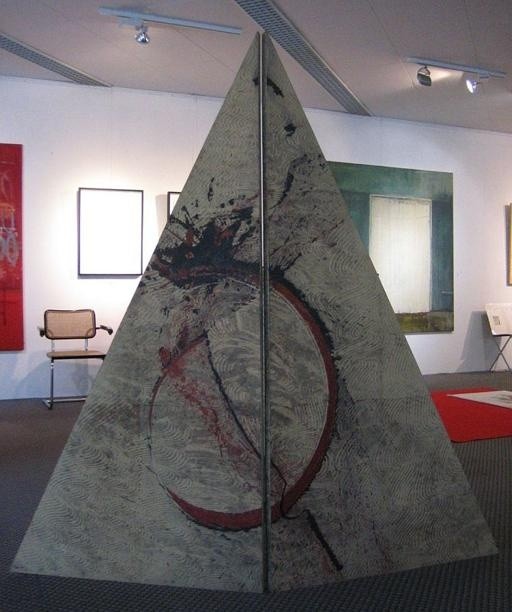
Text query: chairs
38 310 113 409
486 303 512 374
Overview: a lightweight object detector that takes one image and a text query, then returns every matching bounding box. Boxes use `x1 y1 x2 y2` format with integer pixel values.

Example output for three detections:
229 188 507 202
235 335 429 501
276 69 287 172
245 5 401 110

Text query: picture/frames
167 191 183 220
77 188 143 276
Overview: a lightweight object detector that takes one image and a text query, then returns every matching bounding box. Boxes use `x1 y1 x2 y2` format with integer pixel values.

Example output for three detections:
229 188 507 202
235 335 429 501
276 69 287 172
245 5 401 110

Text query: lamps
407 56 506 94
99 8 241 44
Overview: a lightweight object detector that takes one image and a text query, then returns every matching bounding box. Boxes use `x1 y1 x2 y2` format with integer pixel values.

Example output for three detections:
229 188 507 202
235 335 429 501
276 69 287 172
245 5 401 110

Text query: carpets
428 387 512 442
